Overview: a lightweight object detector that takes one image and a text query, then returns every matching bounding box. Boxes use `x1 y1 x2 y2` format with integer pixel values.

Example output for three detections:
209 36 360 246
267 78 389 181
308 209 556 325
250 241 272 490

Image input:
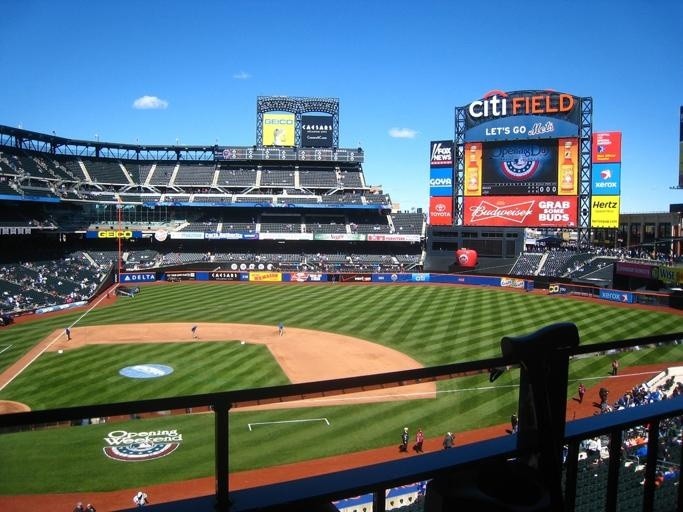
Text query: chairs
559 428 683 512
385 503 424 512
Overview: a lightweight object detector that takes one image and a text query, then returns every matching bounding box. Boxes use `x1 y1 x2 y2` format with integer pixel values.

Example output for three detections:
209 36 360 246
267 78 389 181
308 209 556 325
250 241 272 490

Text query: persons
84 503 96 512
191 326 197 338
400 427 408 452
442 432 453 451
510 412 517 435
562 358 682 489
0 149 416 326
515 236 682 282
414 428 423 453
277 322 283 336
133 492 149 508
72 502 83 511
65 329 71 341
